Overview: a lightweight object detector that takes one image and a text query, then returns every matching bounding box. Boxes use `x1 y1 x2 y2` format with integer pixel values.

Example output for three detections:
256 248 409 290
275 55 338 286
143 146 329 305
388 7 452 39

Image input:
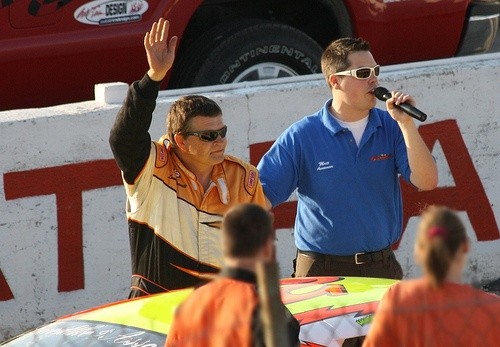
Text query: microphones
374 87 427 122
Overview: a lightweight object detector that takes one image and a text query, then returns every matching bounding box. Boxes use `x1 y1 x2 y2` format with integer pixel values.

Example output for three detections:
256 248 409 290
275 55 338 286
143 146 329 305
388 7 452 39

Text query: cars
0 0 499 113
1 276 400 346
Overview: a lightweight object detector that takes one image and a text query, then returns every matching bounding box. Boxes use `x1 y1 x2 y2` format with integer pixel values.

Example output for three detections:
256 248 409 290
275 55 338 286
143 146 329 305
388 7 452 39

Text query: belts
300 248 390 264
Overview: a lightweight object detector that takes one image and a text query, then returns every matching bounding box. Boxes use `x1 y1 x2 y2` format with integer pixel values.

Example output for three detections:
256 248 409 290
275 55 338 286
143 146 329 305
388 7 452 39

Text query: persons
164 203 301 347
109 17 269 301
256 37 438 280
360 207 500 347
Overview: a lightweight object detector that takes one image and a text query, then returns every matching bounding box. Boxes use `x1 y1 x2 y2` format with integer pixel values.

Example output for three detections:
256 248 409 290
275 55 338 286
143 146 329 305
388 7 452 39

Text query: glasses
175 125 227 142
329 65 380 82
